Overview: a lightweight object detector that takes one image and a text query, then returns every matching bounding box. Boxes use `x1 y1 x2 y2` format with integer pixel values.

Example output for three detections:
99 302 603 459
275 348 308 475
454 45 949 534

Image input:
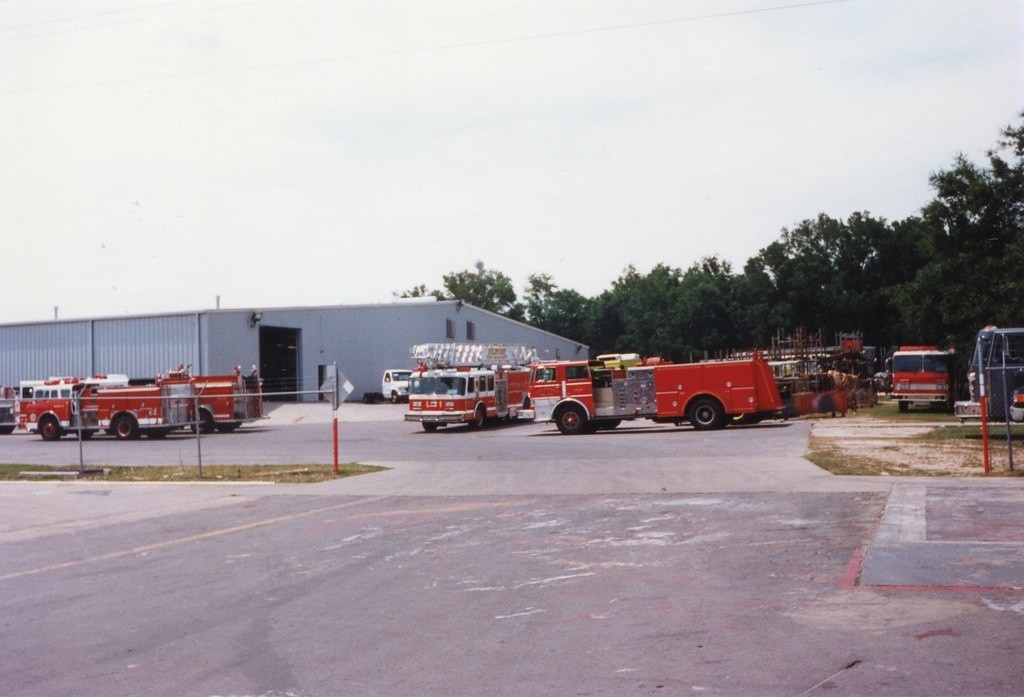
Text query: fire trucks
0 361 271 442
362 343 964 435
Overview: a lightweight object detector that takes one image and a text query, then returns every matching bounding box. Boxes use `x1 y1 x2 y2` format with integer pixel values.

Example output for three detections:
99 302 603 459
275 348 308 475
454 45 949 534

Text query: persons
414 359 427 381
245 365 258 389
884 370 891 397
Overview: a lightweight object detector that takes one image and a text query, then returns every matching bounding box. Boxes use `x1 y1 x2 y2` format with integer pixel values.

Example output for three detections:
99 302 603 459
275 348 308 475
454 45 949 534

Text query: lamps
250 312 262 328
